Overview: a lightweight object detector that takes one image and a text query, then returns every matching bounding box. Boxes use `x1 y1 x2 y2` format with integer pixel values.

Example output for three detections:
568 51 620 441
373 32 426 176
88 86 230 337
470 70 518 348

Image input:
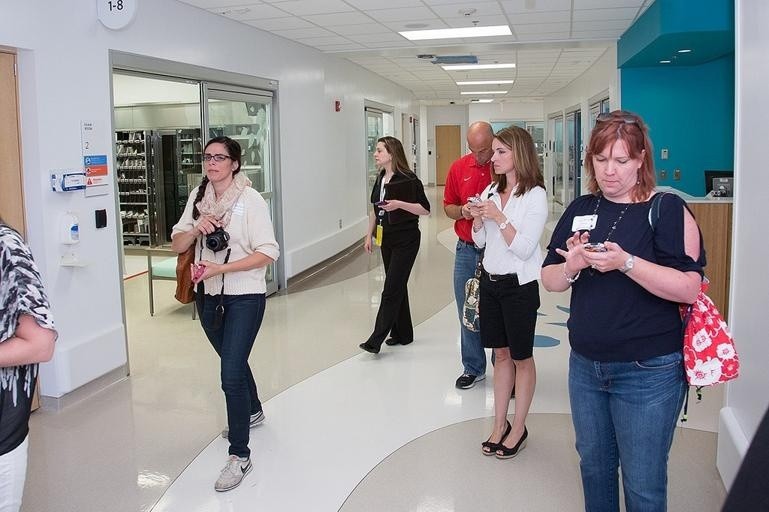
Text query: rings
590 264 596 269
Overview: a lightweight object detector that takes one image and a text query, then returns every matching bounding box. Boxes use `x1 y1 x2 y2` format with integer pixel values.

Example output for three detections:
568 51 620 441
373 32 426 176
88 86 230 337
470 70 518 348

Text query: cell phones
466 196 482 202
374 200 388 206
583 242 607 252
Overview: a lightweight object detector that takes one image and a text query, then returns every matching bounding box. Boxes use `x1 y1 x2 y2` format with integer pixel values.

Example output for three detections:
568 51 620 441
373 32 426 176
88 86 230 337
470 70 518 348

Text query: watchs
498 219 510 231
620 255 634 273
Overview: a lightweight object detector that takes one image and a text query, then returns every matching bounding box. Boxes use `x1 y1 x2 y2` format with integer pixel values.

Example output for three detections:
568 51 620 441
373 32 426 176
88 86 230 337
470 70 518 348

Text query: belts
460 238 474 248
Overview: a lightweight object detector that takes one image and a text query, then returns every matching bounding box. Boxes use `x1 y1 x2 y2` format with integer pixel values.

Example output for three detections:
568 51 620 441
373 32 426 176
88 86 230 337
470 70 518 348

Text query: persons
0 218 59 512
469 124 549 459
171 135 280 492
538 110 706 512
359 136 430 354
444 121 517 398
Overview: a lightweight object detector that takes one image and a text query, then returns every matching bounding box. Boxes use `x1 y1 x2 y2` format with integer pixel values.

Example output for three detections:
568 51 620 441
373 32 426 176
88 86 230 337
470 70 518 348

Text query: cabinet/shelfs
115 124 266 256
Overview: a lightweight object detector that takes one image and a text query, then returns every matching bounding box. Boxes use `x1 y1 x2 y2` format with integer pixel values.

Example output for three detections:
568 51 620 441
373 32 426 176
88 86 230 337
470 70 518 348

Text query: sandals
495 425 528 459
482 419 512 456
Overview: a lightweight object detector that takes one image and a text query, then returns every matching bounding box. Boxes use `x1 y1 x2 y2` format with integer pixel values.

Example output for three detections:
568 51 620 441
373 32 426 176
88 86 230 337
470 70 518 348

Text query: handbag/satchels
462 277 481 332
175 241 196 305
648 191 739 423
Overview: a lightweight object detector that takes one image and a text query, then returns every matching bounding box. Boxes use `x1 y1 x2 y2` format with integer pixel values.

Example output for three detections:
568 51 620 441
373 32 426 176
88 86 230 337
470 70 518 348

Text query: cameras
205 227 231 253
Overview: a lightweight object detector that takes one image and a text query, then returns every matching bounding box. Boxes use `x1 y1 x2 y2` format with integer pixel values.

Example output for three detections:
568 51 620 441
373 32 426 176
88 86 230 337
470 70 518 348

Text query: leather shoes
359 341 381 354
385 336 399 345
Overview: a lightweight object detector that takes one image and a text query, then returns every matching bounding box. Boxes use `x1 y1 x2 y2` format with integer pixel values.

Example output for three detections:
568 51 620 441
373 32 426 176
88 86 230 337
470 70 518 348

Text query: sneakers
456 373 486 389
215 454 253 492
222 410 267 438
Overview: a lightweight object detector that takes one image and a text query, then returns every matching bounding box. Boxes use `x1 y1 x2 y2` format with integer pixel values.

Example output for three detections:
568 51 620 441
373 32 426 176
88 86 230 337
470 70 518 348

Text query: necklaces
588 194 630 277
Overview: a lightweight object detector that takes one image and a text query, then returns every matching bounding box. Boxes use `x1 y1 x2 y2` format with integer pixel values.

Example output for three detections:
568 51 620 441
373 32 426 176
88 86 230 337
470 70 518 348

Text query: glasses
202 153 231 162
595 112 644 134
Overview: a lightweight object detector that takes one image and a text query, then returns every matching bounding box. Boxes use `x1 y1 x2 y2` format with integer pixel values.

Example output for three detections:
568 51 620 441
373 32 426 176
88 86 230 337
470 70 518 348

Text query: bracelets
563 263 581 283
472 223 482 230
